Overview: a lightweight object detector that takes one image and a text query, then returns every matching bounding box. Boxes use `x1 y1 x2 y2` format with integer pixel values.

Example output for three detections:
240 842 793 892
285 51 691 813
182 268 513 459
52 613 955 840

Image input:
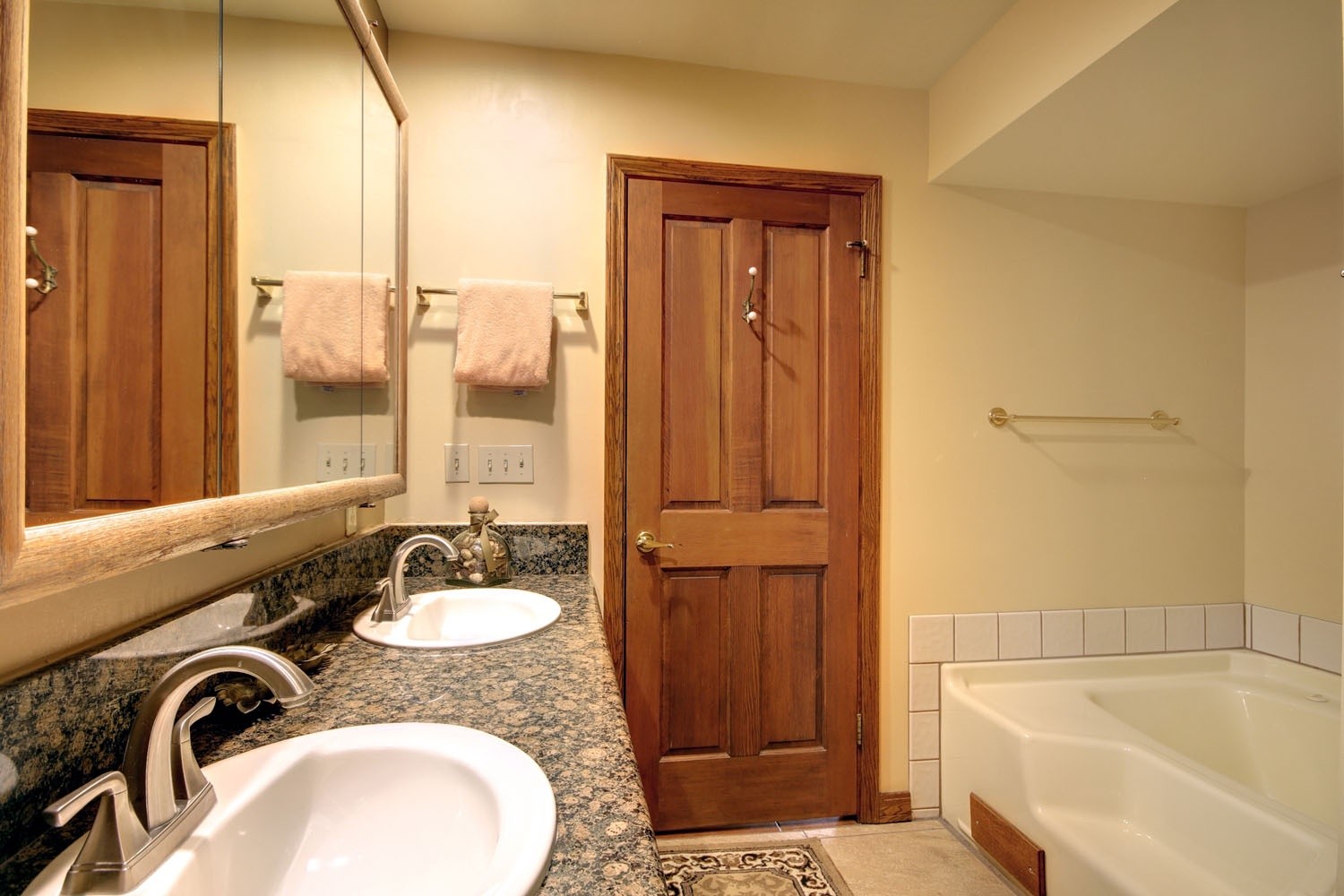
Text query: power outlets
345 506 358 537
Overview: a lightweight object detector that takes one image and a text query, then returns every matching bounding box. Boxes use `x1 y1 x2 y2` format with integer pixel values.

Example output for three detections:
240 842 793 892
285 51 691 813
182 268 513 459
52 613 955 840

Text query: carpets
658 837 854 896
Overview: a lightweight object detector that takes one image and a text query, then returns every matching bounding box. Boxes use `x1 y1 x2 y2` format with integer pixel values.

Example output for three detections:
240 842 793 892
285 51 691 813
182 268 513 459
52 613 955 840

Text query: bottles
442 496 512 587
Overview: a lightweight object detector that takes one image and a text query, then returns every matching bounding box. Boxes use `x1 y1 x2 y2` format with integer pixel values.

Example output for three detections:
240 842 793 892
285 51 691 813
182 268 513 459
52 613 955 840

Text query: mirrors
0 0 409 611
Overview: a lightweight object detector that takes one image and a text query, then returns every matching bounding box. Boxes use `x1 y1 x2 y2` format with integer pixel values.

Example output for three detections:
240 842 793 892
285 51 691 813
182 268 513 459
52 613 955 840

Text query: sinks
91 589 317 662
1 752 19 808
24 721 565 896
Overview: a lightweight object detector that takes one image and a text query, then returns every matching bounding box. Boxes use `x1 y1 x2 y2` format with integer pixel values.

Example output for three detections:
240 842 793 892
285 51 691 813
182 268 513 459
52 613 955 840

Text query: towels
452 276 556 394
281 270 391 395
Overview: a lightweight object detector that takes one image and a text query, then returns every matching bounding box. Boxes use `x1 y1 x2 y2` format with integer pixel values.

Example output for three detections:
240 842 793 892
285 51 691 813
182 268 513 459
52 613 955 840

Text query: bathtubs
936 647 1343 895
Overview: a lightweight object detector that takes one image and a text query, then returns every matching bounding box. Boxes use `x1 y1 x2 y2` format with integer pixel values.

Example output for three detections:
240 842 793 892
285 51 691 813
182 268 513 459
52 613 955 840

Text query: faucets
41 642 321 896
352 586 562 652
370 533 462 623
